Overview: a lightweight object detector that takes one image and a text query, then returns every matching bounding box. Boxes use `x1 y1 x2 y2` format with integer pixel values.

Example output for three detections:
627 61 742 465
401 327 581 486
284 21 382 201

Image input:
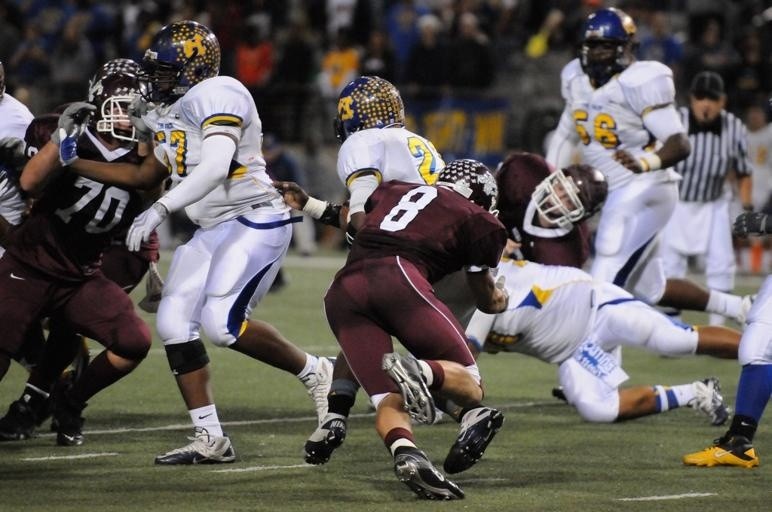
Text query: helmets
88 59 154 143
436 159 499 217
142 20 221 104
334 75 406 141
576 8 636 76
532 164 608 227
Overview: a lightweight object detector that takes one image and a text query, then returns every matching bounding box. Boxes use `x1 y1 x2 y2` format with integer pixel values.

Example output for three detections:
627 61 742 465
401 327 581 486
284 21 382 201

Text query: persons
60 21 336 465
683 192 772 468
324 159 509 503
545 6 754 327
302 76 447 464
0 58 173 447
492 150 608 270
275 181 742 427
1 1 770 291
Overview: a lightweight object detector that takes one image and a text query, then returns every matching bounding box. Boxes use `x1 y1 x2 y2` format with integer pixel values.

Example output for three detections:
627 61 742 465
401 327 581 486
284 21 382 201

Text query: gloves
735 212 767 237
125 203 167 251
0 137 26 171
59 122 86 167
50 102 96 144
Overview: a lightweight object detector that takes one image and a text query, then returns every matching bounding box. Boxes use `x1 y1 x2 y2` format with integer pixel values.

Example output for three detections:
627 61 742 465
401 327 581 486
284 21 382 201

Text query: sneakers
1 370 89 445
156 432 236 464
682 295 759 468
300 353 504 500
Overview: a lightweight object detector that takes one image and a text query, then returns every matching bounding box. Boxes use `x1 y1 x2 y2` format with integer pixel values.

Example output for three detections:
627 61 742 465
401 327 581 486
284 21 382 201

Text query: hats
692 72 723 98
